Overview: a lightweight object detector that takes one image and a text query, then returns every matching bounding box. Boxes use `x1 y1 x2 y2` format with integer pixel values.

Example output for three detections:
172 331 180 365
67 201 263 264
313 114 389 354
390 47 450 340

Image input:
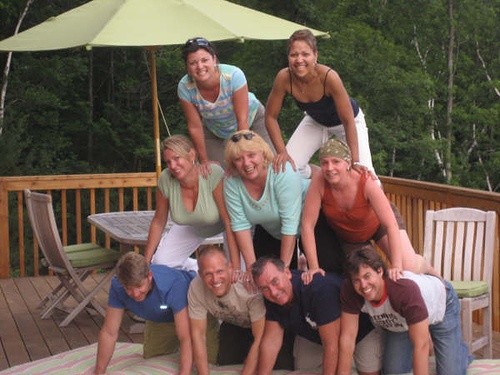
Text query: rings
245 279 250 283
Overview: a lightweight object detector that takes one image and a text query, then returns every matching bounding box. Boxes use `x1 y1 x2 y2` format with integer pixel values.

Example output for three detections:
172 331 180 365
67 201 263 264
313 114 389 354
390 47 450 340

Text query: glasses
185 38 208 47
229 132 256 143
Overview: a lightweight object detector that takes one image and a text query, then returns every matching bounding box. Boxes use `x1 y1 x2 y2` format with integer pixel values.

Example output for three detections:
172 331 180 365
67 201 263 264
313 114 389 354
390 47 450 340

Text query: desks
88 211 225 260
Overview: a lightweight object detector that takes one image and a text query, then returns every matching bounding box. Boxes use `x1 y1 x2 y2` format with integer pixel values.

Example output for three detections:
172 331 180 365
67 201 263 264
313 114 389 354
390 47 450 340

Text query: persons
177 37 277 181
221 129 310 294
142 136 244 284
264 29 386 192
187 245 266 375
251 256 385 375
300 134 443 285
337 247 476 375
94 252 197 375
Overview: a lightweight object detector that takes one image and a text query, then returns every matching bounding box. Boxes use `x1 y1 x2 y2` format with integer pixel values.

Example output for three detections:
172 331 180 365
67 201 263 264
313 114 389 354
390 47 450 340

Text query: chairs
23 189 124 327
423 207 496 360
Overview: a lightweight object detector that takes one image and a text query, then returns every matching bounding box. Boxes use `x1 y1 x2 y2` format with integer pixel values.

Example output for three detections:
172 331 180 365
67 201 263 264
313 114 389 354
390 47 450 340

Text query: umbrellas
0 0 330 183
